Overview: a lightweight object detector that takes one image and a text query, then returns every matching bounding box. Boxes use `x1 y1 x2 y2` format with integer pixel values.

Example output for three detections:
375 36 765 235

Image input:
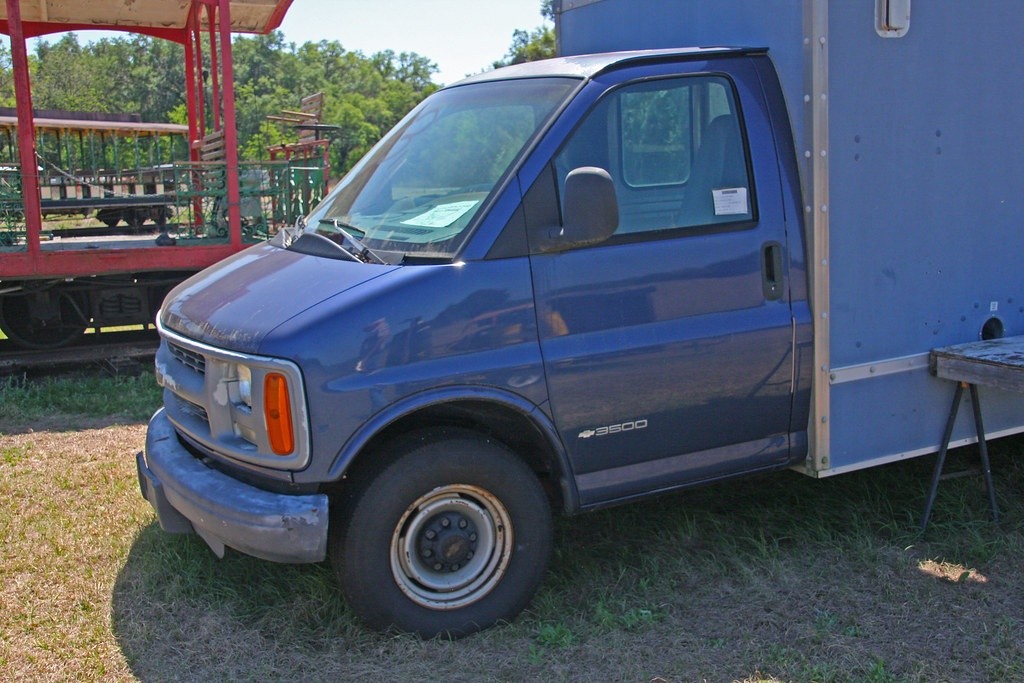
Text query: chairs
535 104 600 171
675 114 752 228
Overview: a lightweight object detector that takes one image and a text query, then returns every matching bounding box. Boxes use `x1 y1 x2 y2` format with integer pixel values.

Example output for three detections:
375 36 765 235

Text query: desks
919 335 1024 538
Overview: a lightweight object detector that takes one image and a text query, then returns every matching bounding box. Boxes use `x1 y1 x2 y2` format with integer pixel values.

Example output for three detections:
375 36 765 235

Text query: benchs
173 129 227 237
265 92 323 151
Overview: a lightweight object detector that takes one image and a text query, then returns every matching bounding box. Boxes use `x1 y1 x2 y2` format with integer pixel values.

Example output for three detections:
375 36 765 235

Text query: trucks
134 0 1024 639
